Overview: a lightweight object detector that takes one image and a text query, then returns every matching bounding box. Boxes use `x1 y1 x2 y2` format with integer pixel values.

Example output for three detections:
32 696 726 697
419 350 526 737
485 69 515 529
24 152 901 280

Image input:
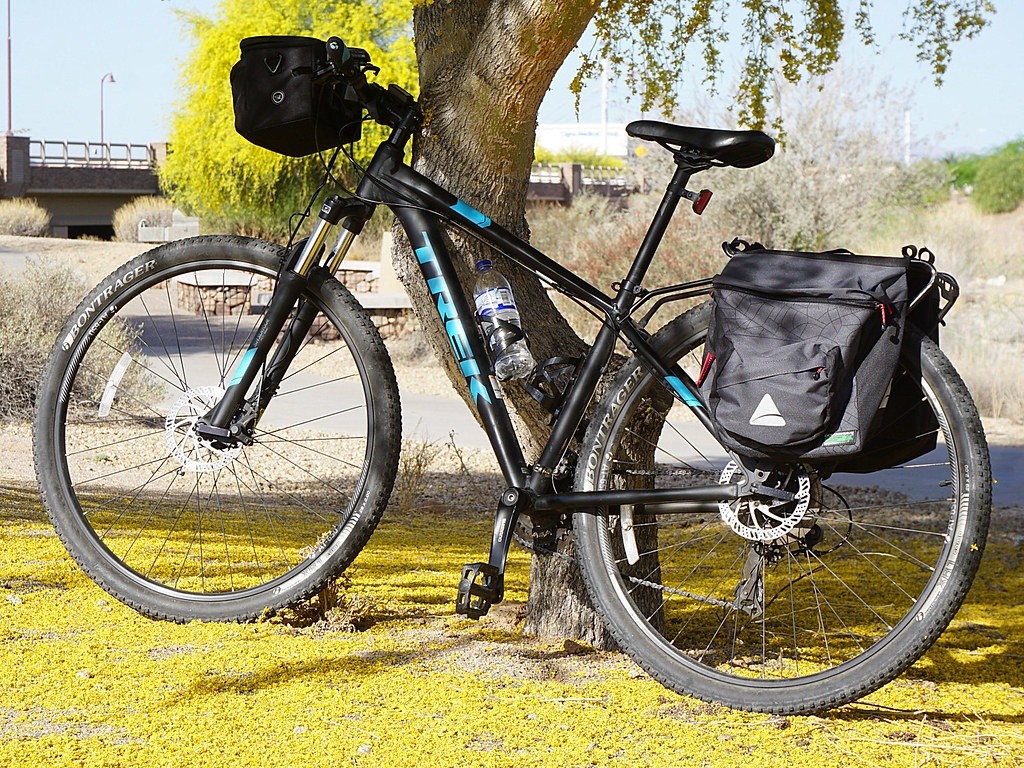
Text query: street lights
100 73 116 167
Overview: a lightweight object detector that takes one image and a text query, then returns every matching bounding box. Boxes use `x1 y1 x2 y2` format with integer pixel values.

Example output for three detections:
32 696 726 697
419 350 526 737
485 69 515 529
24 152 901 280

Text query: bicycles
29 36 995 715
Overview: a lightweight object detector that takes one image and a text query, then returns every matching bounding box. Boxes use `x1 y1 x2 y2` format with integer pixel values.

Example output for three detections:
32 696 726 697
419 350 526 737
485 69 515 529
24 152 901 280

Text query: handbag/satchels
690 250 943 471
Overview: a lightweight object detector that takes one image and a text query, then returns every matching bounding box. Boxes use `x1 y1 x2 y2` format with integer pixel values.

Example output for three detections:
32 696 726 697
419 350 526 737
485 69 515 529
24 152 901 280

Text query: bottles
473 260 535 380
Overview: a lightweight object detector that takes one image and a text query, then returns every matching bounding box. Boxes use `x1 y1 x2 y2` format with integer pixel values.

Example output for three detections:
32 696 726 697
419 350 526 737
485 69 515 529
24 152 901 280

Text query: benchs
258 261 378 293
176 271 258 317
138 219 200 242
258 293 423 344
